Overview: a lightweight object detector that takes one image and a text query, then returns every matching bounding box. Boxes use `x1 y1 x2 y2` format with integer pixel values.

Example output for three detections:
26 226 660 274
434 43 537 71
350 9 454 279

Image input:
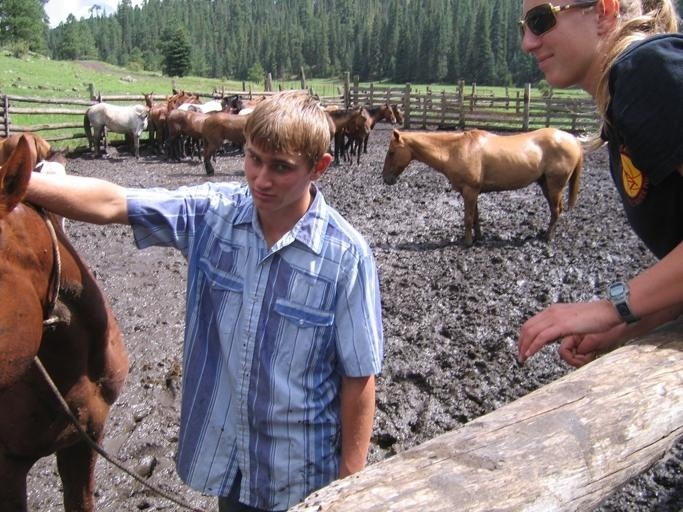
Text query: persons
24 90 385 512
518 0 683 365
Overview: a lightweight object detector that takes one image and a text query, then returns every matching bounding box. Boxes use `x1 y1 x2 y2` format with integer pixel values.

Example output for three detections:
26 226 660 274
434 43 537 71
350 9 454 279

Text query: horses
382 127 585 248
84 90 403 177
0 131 131 512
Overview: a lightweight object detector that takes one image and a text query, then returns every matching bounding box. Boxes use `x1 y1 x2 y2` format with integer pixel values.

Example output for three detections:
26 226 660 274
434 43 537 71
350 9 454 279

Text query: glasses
515 1 598 42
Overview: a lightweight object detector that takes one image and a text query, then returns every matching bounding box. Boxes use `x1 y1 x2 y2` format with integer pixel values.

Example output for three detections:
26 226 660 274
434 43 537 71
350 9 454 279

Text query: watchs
605 279 639 327
